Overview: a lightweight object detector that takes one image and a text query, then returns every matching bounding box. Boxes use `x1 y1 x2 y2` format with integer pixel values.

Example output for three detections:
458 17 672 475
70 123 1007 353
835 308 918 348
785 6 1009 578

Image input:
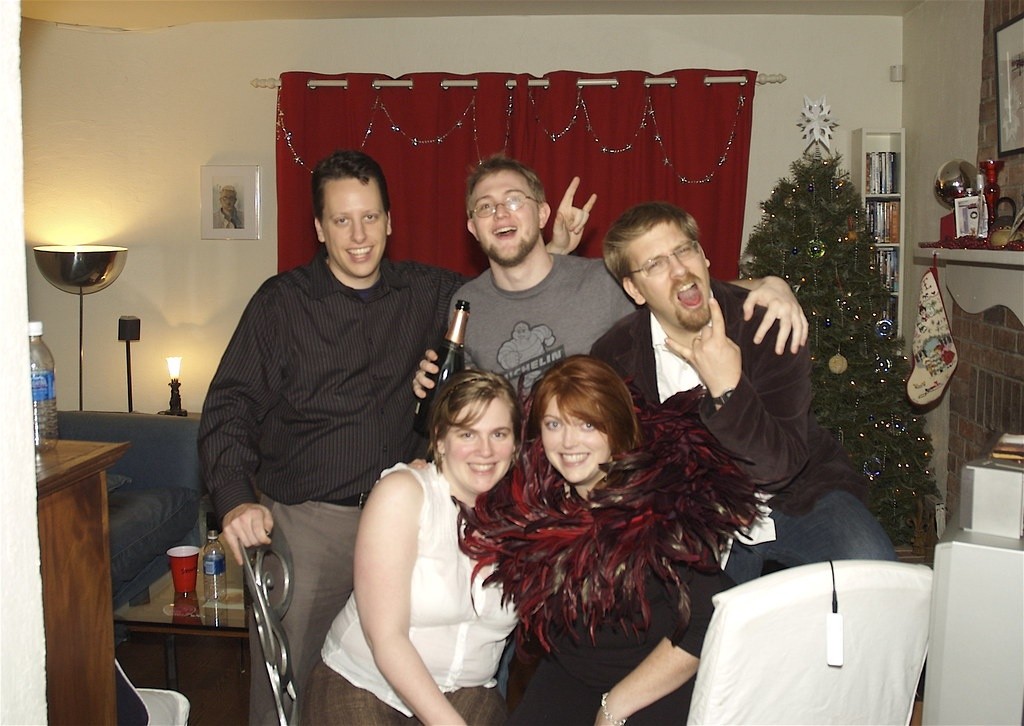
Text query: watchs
712 387 735 406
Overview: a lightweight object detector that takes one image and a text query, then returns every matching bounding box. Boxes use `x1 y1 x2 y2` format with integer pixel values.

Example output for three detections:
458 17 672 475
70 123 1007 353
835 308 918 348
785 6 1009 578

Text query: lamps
33 246 128 411
165 356 188 416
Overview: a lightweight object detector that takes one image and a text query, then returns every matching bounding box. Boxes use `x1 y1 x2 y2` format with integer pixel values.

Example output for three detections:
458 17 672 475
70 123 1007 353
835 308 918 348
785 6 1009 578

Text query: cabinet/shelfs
853 127 906 342
33 439 134 726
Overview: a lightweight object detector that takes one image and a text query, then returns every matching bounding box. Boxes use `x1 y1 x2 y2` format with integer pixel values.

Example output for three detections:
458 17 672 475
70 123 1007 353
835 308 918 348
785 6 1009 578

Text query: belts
328 490 372 511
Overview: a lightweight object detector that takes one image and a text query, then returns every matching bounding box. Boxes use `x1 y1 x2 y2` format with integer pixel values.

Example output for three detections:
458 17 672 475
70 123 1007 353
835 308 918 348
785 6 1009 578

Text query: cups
166 546 201 593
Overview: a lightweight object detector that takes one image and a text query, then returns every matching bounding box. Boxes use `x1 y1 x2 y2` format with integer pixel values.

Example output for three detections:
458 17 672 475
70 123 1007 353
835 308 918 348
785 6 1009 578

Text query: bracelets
601 693 626 726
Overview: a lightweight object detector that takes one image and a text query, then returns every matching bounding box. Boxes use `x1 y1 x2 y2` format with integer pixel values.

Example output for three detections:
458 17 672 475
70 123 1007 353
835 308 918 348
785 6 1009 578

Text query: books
866 201 899 244
864 248 899 292
866 152 898 194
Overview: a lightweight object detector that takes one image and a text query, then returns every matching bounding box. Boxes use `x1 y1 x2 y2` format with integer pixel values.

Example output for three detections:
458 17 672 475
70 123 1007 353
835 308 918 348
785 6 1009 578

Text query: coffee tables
112 531 250 691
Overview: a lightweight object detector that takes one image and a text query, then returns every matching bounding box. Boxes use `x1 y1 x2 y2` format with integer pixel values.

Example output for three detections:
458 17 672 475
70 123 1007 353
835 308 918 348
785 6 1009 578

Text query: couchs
53 411 202 611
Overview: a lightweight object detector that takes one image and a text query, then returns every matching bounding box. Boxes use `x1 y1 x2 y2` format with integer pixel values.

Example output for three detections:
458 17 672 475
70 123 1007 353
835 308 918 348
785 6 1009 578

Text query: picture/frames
200 164 261 242
993 12 1024 158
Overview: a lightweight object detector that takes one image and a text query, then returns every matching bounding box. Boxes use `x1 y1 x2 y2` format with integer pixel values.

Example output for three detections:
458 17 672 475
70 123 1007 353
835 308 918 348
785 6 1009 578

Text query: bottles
411 298 472 439
28 320 60 452
203 530 228 603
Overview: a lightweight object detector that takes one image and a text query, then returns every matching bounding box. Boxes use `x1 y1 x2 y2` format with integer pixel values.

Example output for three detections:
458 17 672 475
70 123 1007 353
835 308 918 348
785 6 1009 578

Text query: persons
496 354 739 726
414 151 808 401
301 367 527 726
588 200 903 585
213 186 244 229
196 147 598 726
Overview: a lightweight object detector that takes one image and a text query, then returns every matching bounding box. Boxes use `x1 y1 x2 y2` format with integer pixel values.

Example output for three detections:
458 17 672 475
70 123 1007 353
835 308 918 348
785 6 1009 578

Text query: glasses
470 193 541 218
626 240 699 277
